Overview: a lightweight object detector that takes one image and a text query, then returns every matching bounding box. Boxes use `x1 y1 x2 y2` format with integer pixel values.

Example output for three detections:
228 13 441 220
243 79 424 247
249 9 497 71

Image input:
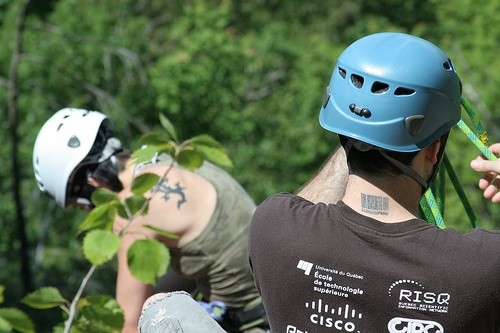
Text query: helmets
318 30 464 152
32 107 112 210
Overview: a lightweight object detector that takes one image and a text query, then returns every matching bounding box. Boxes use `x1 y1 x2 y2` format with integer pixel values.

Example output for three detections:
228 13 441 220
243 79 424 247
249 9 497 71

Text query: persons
246 31 500 332
32 107 271 333
